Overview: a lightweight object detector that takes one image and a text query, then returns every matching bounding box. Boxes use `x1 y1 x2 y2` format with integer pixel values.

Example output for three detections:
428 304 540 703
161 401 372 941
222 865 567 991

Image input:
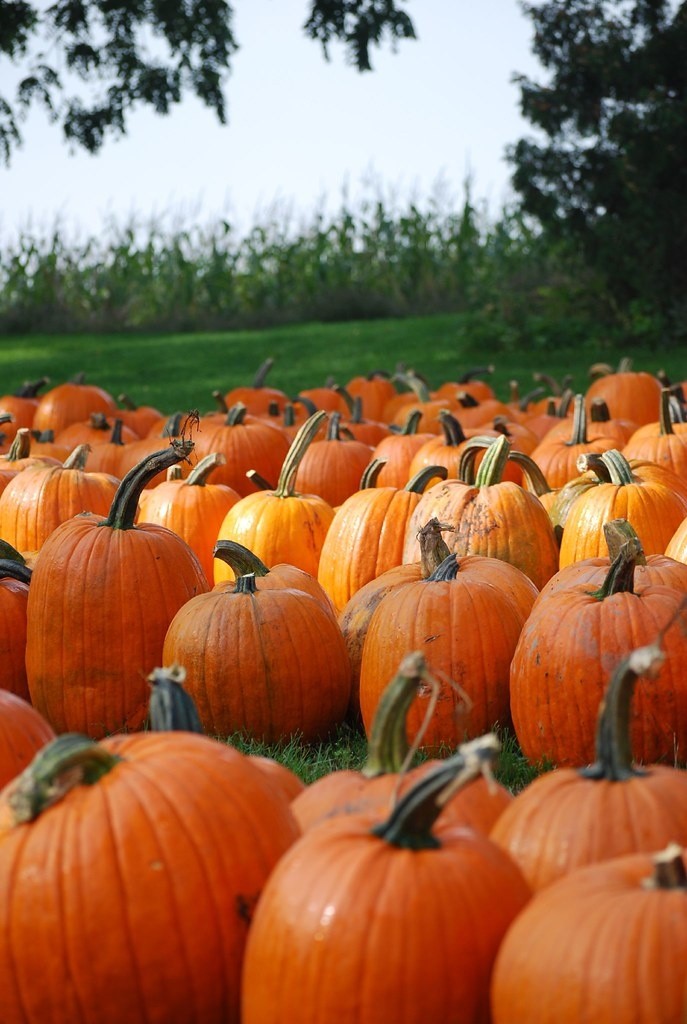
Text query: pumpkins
0 360 687 1024
